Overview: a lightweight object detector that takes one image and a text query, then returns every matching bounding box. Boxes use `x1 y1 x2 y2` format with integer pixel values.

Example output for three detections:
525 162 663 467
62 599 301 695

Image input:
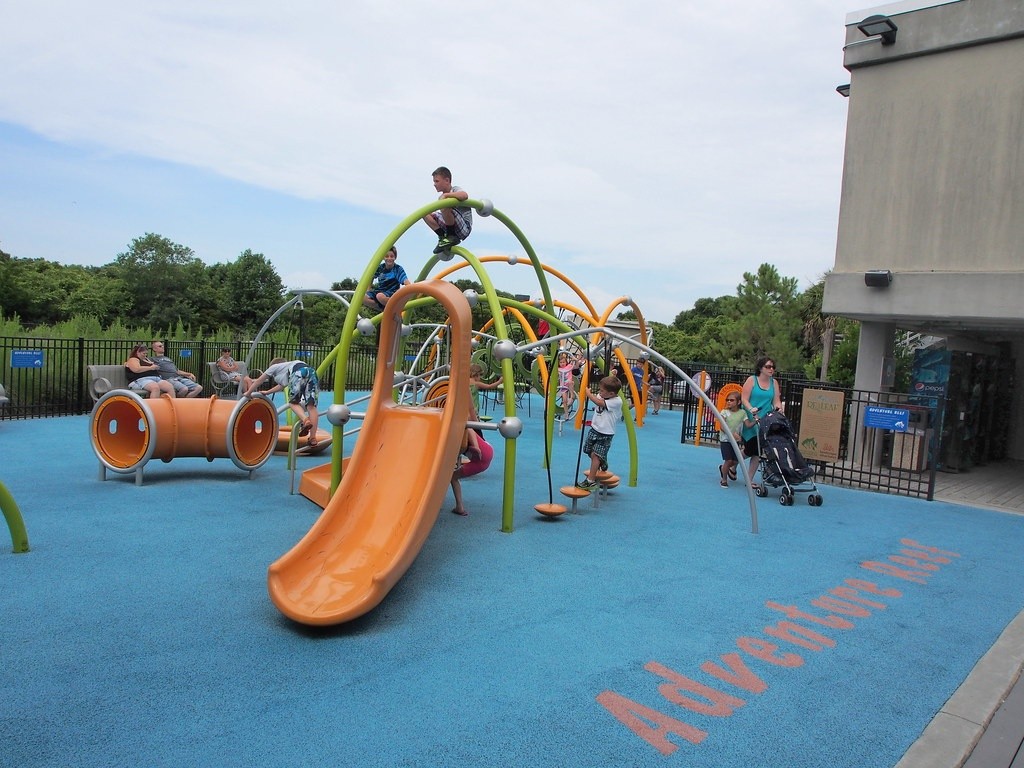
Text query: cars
670 381 702 405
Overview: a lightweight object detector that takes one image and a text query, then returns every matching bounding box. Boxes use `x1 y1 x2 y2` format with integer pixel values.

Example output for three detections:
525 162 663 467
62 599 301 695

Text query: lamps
836 84 850 97
858 15 898 46
865 270 892 287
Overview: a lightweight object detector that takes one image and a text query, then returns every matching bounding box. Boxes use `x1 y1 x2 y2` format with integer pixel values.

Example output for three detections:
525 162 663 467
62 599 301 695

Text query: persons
577 376 623 490
650 366 665 415
362 246 411 311
469 365 503 441
621 358 646 425
558 356 579 421
244 357 319 446
728 356 784 489
718 391 757 489
422 166 472 254
610 360 618 376
124 341 203 398
125 344 176 399
216 346 252 393
451 427 493 516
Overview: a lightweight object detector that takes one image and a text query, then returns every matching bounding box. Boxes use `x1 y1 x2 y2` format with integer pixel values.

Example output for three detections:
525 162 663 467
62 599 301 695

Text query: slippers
452 507 468 516
727 468 737 480
745 483 759 489
469 447 482 461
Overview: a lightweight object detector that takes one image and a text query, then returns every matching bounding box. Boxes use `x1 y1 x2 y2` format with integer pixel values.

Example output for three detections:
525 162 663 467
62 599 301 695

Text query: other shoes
652 410 658 414
633 418 645 424
567 399 572 407
565 413 569 420
718 464 723 479
439 234 461 248
433 238 444 254
719 481 728 488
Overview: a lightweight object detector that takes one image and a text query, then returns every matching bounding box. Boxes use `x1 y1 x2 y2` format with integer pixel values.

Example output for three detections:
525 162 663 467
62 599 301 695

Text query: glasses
726 398 736 402
155 345 163 348
222 349 230 353
763 364 775 370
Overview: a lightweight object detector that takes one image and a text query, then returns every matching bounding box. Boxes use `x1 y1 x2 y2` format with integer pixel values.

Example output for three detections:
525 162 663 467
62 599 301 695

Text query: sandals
307 438 318 446
299 418 313 437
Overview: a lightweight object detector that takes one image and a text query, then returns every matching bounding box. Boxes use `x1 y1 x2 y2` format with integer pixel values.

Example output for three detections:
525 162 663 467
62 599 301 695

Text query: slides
265 280 471 622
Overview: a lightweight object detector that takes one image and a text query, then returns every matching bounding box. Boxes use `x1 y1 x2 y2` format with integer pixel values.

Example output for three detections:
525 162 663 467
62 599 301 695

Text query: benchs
207 361 269 399
86 364 178 404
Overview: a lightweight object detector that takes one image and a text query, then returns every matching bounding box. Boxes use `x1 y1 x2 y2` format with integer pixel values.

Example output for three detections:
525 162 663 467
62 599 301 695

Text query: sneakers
576 478 598 490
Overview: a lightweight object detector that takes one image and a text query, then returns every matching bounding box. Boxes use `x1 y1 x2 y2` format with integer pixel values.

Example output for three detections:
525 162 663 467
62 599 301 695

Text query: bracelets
749 407 753 410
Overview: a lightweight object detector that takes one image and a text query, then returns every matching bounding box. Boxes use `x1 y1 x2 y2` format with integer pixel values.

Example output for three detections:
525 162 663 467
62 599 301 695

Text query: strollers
752 405 826 510
